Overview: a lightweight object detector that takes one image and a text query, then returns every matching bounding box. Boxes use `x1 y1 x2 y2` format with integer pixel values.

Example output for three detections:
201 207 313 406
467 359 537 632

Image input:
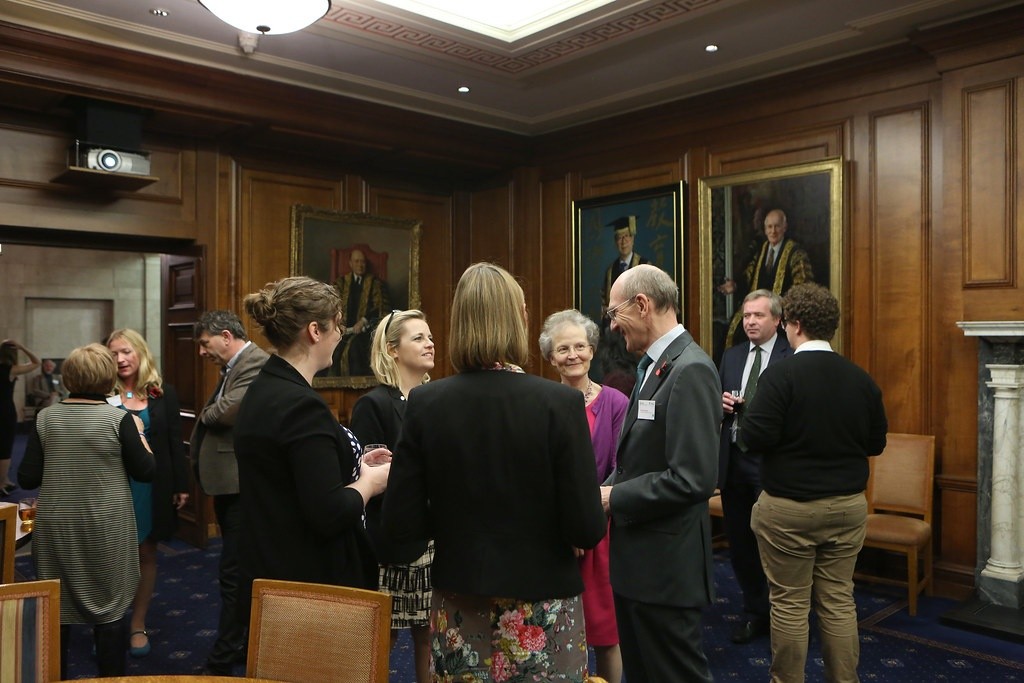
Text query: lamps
196 0 333 35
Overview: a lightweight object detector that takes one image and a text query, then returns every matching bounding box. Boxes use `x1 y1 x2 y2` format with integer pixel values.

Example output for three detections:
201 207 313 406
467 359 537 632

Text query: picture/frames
571 179 689 383
699 154 847 370
289 202 422 392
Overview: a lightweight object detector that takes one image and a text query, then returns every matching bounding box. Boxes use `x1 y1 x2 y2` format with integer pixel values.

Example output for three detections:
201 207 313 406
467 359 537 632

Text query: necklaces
585 378 593 402
121 388 139 398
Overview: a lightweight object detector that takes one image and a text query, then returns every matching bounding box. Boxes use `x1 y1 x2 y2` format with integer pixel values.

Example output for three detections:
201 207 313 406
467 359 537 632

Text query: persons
600 214 653 335
322 249 392 377
0 264 889 683
719 208 814 350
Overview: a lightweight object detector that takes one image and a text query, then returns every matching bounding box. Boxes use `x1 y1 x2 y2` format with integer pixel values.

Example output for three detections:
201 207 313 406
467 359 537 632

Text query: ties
620 263 626 272
766 248 773 277
621 353 653 443
743 347 761 411
356 277 360 284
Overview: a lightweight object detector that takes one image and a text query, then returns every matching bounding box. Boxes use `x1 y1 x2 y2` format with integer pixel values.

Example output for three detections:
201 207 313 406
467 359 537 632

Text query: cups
364 444 388 467
19 497 36 526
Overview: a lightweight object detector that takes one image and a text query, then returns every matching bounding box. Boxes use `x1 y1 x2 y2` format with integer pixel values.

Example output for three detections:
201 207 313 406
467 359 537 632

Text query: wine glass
729 391 744 430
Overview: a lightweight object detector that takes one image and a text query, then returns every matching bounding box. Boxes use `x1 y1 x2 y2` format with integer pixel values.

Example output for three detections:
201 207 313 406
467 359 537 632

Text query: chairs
245 579 393 683
0 579 61 683
852 431 937 618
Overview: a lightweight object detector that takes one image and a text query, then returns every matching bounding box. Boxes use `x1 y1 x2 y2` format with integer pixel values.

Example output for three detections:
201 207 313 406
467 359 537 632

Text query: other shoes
129 631 150 657
730 615 770 644
195 664 232 677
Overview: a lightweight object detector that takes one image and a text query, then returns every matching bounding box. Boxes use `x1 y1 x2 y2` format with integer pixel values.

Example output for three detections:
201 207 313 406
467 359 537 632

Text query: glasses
607 294 650 321
385 309 401 335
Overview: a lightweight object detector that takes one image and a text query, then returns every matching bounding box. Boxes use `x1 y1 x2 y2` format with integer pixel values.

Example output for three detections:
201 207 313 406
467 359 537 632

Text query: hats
604 215 640 231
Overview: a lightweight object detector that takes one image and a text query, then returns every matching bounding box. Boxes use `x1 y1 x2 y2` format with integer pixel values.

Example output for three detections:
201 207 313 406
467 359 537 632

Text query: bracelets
139 432 145 436
